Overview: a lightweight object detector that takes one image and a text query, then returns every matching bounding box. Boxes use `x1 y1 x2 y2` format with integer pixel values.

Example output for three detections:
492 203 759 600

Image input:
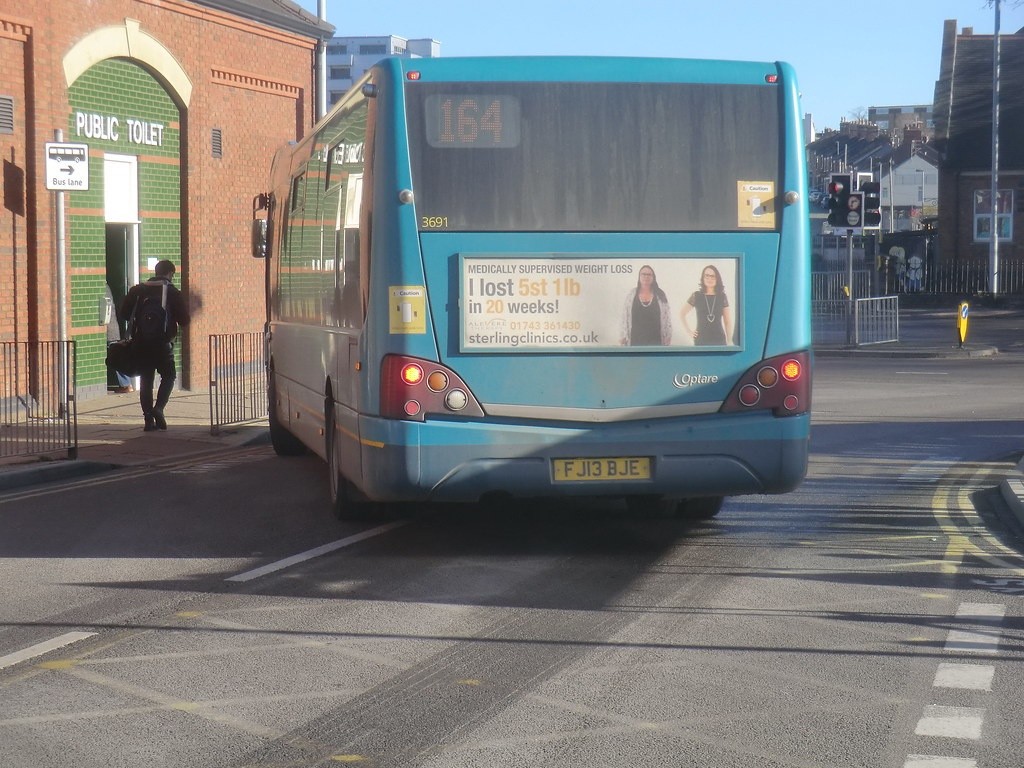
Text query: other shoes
151 406 166 429
114 385 133 393
144 420 156 431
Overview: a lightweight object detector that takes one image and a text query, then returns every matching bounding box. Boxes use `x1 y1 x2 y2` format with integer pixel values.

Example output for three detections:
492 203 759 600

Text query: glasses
639 273 654 279
703 274 718 280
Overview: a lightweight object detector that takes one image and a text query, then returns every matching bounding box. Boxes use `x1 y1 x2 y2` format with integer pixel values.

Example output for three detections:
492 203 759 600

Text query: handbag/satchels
104 338 136 377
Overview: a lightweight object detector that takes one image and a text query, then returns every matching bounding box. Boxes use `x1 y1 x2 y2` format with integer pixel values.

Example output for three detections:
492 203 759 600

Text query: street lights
915 168 926 227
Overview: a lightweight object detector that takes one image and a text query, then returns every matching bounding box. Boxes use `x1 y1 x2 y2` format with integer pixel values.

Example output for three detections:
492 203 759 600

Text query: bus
251 54 814 521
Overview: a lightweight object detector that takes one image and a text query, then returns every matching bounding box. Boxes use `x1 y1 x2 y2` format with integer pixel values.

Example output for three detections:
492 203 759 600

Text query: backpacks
128 284 171 355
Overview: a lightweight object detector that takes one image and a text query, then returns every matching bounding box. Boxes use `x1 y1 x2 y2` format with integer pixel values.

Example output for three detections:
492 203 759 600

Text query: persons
618 265 674 346
107 260 191 432
679 265 735 346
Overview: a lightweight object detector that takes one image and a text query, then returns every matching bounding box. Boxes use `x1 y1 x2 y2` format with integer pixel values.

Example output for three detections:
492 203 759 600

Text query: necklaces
638 292 654 307
704 293 716 322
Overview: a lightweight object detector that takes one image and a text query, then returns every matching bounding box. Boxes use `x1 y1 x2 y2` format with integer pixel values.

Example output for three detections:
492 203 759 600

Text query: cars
809 187 828 209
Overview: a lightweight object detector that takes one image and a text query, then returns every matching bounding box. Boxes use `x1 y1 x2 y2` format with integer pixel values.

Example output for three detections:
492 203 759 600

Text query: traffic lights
827 173 853 231
858 173 882 230
